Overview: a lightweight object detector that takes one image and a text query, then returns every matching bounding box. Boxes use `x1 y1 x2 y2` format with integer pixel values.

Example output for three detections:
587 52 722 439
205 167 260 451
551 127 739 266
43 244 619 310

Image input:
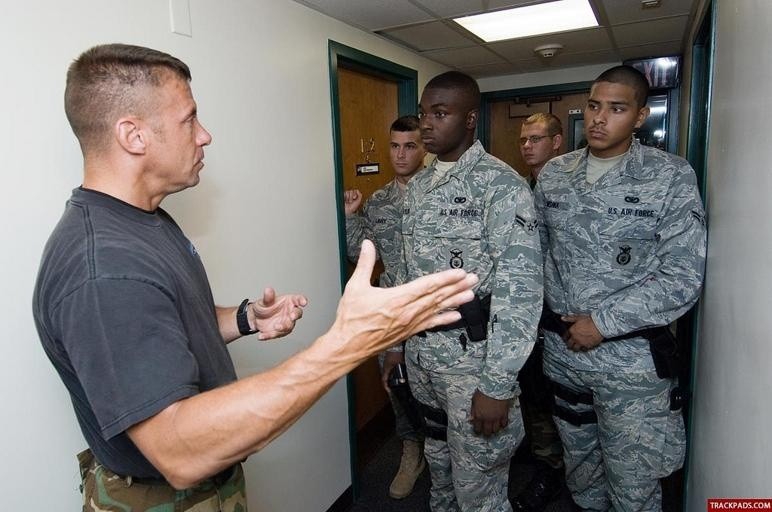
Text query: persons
343 116 430 499
32 43 481 512
514 110 565 511
380 71 545 512
532 65 708 511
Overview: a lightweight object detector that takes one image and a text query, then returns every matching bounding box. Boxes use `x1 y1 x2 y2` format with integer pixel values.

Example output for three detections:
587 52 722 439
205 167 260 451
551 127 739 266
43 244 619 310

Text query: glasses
521 137 546 145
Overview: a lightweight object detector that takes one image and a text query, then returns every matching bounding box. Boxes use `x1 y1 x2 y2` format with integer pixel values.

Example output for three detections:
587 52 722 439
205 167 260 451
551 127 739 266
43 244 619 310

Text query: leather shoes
513 472 566 511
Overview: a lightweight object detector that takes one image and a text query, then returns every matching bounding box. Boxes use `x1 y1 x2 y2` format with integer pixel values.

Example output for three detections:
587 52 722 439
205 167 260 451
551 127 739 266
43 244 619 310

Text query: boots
390 440 426 499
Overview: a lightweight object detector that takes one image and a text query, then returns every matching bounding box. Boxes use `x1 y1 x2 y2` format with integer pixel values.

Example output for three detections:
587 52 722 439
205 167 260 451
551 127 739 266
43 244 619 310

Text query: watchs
236 299 259 336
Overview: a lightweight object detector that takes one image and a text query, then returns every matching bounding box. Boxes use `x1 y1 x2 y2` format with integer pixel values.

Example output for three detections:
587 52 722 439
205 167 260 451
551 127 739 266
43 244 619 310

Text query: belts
109 471 168 487
